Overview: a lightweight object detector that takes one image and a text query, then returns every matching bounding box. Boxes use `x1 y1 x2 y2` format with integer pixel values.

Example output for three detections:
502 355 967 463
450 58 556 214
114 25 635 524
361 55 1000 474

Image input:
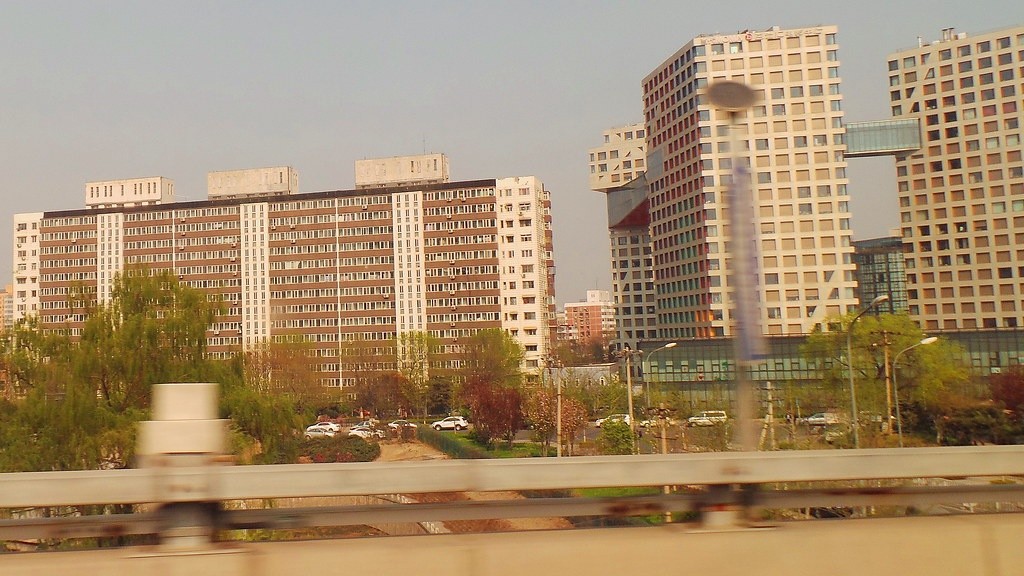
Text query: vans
689 411 727 428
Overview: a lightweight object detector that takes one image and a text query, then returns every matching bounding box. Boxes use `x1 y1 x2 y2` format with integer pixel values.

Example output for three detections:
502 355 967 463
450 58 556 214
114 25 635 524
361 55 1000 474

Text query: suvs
801 413 838 426
432 416 468 431
596 414 630 428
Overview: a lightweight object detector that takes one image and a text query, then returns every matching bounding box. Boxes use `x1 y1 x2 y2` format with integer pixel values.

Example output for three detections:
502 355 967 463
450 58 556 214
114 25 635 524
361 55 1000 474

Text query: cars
349 428 386 439
641 420 657 427
388 420 417 429
850 411 897 434
351 421 375 429
303 428 334 439
306 421 342 433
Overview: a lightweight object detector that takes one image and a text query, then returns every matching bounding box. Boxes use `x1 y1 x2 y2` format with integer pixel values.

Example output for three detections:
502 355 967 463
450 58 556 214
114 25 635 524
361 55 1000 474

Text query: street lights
646 343 677 418
847 295 889 515
893 337 939 447
